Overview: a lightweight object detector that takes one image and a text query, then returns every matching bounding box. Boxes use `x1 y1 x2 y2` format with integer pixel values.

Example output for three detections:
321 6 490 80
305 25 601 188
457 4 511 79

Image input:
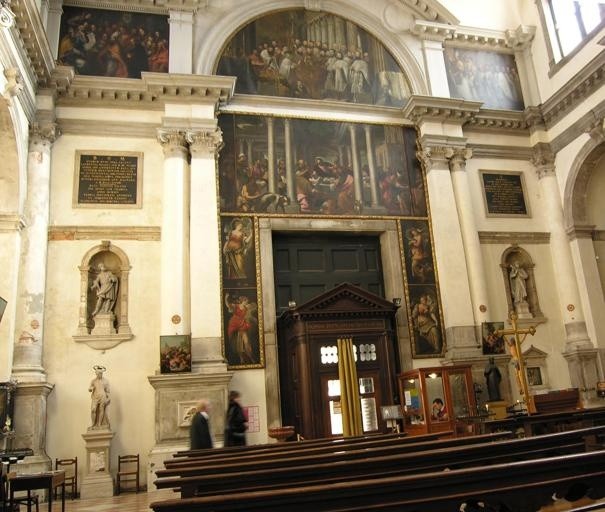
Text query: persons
234 152 423 215
89 262 119 316
449 57 523 110
190 398 214 448
483 355 503 399
224 391 249 446
405 226 431 283
221 220 253 277
58 11 168 78
509 260 530 303
247 37 373 103
224 292 258 363
410 294 442 352
87 364 111 426
431 398 448 421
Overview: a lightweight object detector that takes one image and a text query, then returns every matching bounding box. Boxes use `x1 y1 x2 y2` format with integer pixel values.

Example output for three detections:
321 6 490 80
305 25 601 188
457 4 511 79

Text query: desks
10 471 66 512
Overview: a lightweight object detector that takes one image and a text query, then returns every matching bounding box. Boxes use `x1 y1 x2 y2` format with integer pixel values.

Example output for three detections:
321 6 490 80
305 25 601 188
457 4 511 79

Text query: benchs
149 407 605 512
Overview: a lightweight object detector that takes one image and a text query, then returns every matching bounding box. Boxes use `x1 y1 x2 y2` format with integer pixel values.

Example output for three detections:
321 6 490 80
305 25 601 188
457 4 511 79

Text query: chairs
117 453 140 495
54 457 77 501
0 458 39 512
